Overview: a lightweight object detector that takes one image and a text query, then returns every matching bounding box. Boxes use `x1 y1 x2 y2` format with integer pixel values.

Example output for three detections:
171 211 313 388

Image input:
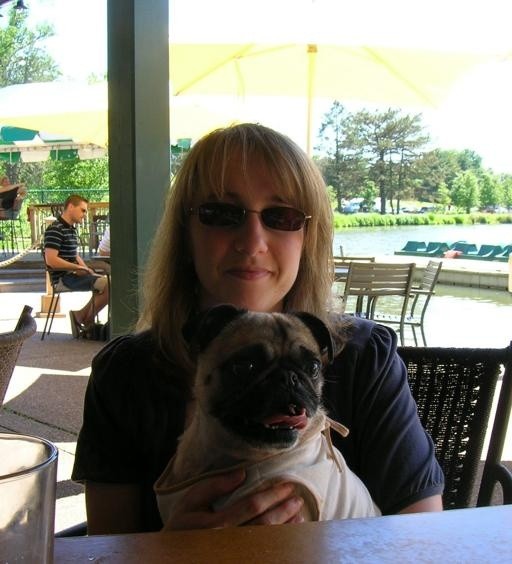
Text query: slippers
69 311 81 339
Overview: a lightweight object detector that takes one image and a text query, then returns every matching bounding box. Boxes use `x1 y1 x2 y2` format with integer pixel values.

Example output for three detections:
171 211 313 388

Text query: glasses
188 199 314 231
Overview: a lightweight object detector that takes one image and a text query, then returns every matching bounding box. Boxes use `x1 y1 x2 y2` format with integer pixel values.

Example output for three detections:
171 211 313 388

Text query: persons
43 194 111 339
70 126 447 537
98 225 111 256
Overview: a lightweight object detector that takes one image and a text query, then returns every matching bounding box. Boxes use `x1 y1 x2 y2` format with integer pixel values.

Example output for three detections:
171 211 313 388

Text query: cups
0 432 59 564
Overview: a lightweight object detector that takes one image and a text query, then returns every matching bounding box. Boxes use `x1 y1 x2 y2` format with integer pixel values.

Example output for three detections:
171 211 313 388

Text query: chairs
2 305 37 406
334 257 442 348
41 240 111 339
0 182 28 257
396 341 512 510
50 203 86 257
90 215 107 255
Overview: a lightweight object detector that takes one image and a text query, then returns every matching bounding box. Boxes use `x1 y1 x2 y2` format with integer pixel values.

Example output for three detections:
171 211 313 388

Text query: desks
91 254 111 275
29 202 110 251
52 504 512 562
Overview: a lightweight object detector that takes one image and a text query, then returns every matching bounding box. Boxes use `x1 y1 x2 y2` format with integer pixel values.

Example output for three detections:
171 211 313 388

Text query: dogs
158 302 382 531
